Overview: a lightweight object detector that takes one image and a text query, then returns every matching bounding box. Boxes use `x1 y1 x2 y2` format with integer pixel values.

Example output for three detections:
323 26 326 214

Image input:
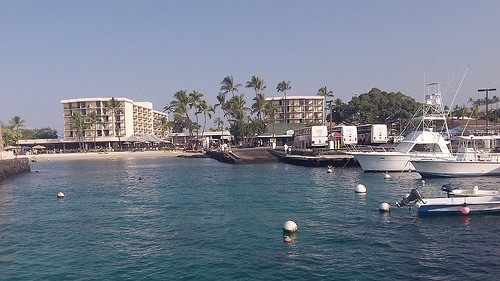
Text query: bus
330 126 357 145
294 126 328 147
356 124 387 145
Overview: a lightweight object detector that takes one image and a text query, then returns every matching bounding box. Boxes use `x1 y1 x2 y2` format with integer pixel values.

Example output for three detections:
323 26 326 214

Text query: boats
346 132 500 171
396 188 500 214
441 182 500 197
408 136 500 177
